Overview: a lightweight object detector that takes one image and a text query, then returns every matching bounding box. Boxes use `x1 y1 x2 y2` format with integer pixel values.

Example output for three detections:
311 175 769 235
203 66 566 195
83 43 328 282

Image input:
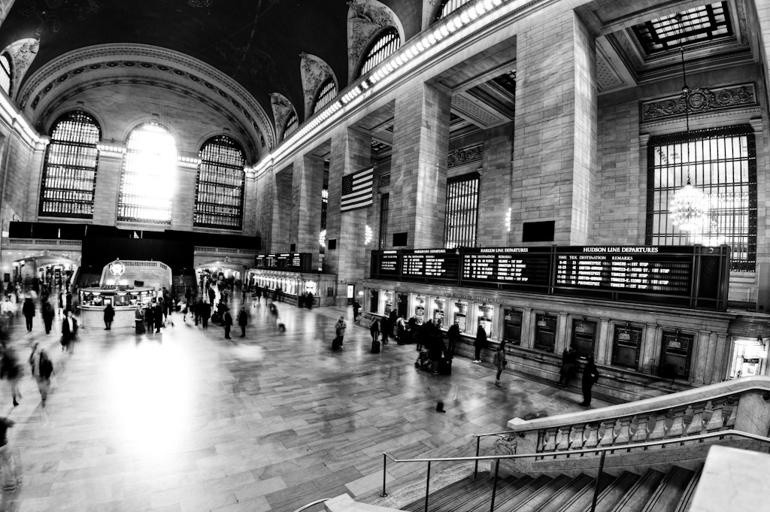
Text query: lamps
672 11 711 235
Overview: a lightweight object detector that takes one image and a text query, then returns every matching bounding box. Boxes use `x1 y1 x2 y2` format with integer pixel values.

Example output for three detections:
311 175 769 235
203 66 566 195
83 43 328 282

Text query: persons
471 325 488 364
185 269 284 339
79 291 103 306
352 299 361 322
1 346 24 407
334 316 346 351
579 356 599 407
59 310 79 355
558 344 576 387
1 417 19 489
29 342 54 408
103 304 115 330
495 339 505 387
368 307 461 378
125 285 180 333
303 292 315 310
5 269 72 334
